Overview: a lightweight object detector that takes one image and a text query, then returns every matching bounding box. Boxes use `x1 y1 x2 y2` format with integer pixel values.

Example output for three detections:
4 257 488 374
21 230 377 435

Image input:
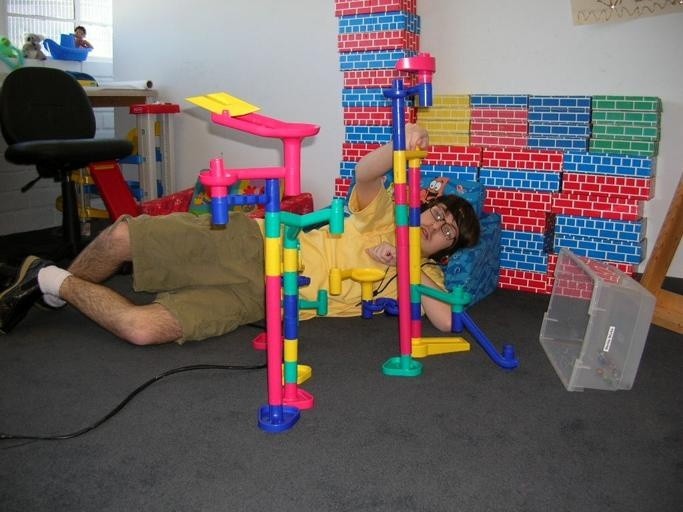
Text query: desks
82 84 157 203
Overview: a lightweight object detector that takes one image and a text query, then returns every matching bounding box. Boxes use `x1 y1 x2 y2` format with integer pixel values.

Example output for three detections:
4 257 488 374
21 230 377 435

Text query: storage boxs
538 250 657 393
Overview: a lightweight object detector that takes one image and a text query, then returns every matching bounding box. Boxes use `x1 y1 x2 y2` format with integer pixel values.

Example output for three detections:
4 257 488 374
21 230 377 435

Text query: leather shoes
0 255 67 334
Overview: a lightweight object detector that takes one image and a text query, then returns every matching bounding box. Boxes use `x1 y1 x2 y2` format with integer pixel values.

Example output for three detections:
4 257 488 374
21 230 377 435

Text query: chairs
0 67 134 274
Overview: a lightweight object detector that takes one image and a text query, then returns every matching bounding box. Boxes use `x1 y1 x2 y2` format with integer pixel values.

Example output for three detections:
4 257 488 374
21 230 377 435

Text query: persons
0 124 480 346
75 26 93 48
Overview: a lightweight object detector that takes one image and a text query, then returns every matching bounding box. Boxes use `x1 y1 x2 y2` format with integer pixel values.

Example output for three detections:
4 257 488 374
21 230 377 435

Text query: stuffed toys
0 33 46 69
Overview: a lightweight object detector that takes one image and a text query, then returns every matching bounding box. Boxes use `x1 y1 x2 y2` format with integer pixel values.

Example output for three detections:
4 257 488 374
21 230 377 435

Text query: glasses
430 205 457 240
